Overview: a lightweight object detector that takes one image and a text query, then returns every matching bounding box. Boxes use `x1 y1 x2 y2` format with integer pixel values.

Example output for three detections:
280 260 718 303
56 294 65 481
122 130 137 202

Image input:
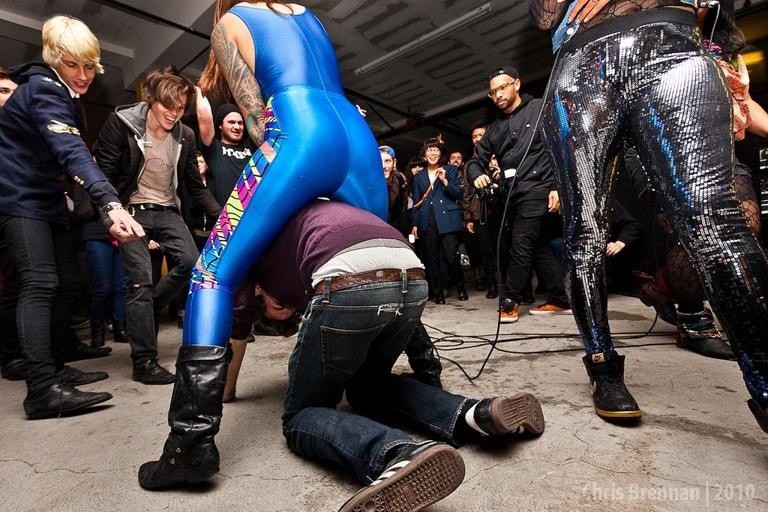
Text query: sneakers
465 392 546 439
498 302 520 323
337 440 467 511
529 303 574 316
582 345 642 419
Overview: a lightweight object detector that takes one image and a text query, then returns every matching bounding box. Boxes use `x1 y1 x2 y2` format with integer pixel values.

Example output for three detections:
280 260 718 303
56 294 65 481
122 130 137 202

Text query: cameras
475 185 499 202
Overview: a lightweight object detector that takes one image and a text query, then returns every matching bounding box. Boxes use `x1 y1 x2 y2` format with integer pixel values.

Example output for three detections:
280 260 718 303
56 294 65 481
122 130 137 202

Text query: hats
216 101 241 124
379 145 396 159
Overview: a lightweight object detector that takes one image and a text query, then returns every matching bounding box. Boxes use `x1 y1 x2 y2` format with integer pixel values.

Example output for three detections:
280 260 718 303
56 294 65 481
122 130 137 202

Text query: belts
126 198 182 215
313 267 428 296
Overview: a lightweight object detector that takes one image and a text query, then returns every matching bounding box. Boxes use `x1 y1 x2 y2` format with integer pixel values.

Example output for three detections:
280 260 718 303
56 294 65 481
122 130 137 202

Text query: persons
0 12 149 423
134 2 445 494
528 0 766 435
0 62 768 387
218 198 547 512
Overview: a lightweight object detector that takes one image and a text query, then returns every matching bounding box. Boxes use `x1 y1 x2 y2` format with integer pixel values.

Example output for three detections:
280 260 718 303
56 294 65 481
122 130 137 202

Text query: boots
675 311 738 360
139 344 233 495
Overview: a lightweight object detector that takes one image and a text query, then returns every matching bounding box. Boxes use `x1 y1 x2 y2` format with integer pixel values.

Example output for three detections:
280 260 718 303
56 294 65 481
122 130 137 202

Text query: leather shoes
457 290 470 301
68 343 116 361
435 293 446 303
486 287 499 300
56 364 109 385
24 387 111 418
131 354 176 385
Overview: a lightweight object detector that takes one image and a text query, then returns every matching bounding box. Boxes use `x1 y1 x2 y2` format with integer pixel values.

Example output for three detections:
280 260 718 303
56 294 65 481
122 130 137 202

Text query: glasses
487 80 516 97
